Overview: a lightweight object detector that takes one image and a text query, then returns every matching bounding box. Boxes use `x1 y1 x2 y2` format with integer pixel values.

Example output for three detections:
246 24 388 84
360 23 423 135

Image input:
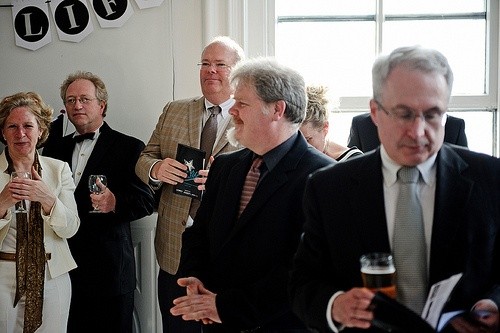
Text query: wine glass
11 171 32 213
89 175 107 213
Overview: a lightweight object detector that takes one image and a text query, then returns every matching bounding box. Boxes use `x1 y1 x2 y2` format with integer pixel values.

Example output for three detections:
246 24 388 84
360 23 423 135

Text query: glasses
197 62 230 68
374 100 446 125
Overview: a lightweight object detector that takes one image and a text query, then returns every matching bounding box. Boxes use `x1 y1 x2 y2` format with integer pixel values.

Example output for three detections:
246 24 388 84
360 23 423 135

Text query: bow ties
74 132 95 143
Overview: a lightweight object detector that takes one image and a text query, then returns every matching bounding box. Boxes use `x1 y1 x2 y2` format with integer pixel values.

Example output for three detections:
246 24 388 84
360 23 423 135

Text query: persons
170 59 339 333
296 46 500 333
348 112 468 155
43 71 154 333
299 84 365 162
0 92 80 333
135 36 249 333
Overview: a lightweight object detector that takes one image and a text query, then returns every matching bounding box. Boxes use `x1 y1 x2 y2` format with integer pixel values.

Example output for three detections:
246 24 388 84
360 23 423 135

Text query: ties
393 166 428 316
189 106 221 221
238 157 262 218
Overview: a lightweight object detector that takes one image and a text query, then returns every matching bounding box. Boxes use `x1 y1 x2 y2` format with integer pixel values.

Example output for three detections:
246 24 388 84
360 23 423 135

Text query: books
173 143 207 201
365 273 488 333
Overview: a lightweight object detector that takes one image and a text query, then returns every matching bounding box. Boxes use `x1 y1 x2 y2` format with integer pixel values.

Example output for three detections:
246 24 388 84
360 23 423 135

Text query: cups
360 253 397 299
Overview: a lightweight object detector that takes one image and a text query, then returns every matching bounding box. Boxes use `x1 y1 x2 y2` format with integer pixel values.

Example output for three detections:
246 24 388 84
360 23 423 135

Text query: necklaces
323 140 330 156
